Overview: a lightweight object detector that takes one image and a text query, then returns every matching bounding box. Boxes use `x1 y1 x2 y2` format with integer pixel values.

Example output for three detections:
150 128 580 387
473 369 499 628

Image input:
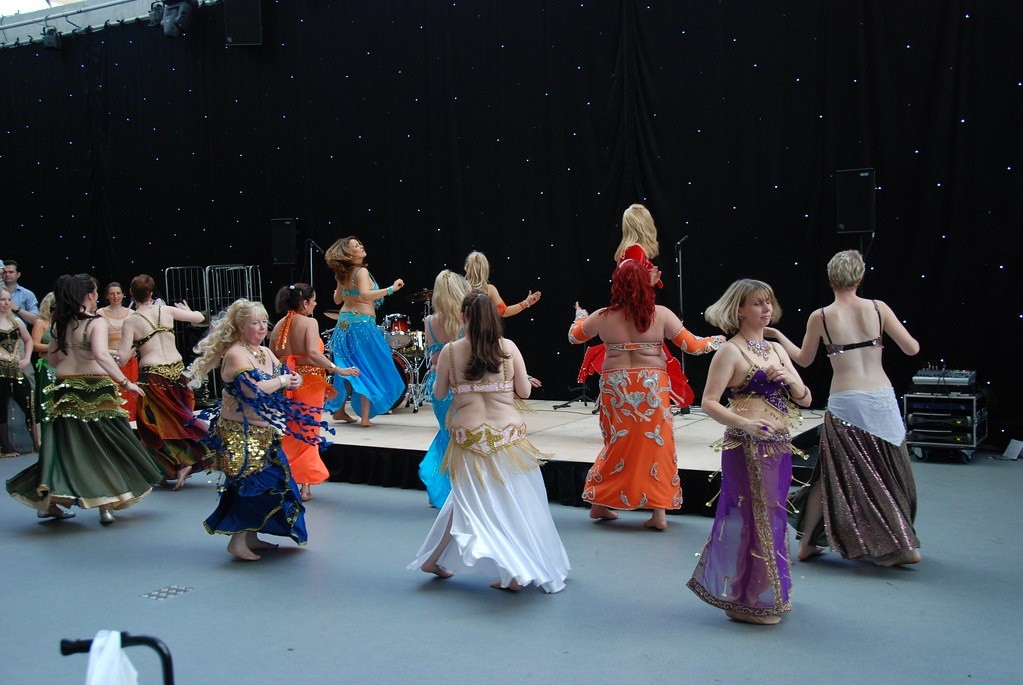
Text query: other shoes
38 505 75 519
100 510 116 523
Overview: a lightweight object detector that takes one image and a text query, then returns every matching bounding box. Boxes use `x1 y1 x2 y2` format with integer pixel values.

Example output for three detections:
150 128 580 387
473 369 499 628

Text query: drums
383 313 412 349
382 349 414 415
393 331 425 357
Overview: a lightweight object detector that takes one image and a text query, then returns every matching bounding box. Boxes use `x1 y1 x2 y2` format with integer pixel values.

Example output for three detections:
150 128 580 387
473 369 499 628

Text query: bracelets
326 363 337 374
117 378 131 390
518 303 525 310
277 376 287 388
387 286 394 296
15 307 22 313
523 300 530 308
795 387 808 400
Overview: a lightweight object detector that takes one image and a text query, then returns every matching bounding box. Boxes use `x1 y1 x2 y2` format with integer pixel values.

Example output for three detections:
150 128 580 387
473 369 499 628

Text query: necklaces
738 331 770 360
238 337 266 365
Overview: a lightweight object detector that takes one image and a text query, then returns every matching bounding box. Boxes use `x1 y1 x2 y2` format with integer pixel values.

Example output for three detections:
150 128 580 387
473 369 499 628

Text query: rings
352 366 355 369
760 426 768 431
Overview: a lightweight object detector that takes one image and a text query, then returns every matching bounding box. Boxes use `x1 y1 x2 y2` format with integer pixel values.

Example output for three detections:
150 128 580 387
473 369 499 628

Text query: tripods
553 380 597 410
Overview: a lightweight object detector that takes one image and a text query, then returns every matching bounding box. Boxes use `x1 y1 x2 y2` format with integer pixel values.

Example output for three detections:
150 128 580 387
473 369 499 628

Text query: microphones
677 235 688 246
312 241 324 253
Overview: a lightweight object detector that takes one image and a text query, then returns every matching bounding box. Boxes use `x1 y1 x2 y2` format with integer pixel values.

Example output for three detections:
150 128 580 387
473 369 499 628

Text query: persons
187 298 308 561
418 291 572 594
567 259 727 530
613 203 663 287
268 282 360 502
765 250 923 568
685 278 812 625
462 251 541 318
0 260 219 525
322 236 406 426
417 269 542 510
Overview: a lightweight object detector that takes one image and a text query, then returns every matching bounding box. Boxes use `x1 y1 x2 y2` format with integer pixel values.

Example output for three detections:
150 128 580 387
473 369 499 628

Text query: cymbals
323 309 339 320
405 290 433 300
